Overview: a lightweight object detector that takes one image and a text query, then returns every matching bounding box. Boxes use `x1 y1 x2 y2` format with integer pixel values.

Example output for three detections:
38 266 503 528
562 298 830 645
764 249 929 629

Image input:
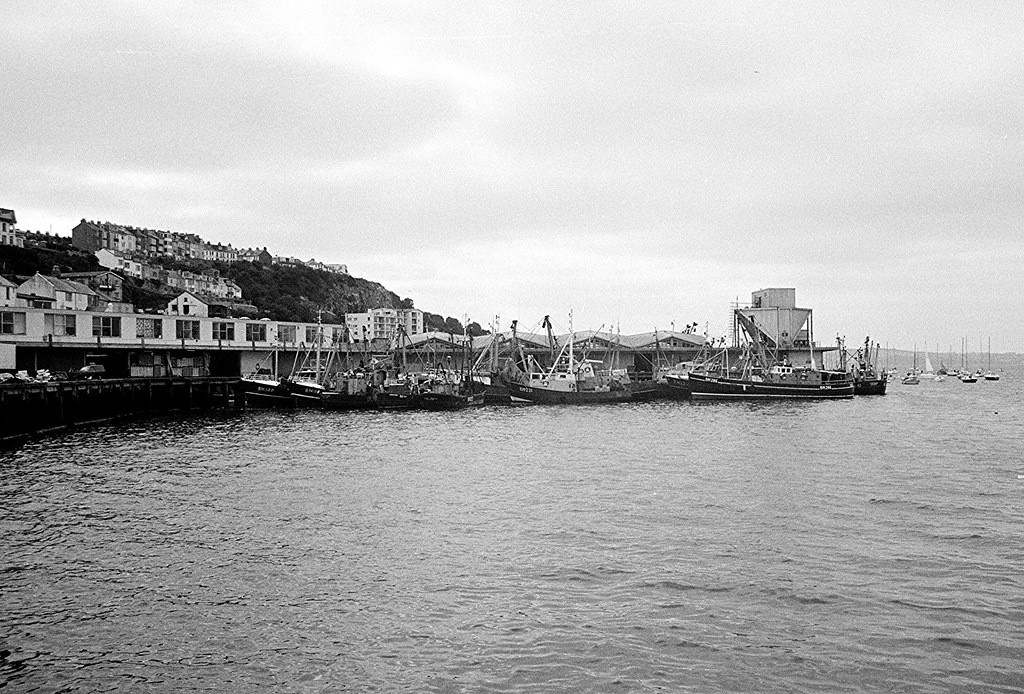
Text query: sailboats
236 309 1001 412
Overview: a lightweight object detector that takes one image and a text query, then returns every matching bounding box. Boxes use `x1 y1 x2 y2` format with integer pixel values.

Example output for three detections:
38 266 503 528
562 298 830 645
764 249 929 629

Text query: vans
75 364 107 379
